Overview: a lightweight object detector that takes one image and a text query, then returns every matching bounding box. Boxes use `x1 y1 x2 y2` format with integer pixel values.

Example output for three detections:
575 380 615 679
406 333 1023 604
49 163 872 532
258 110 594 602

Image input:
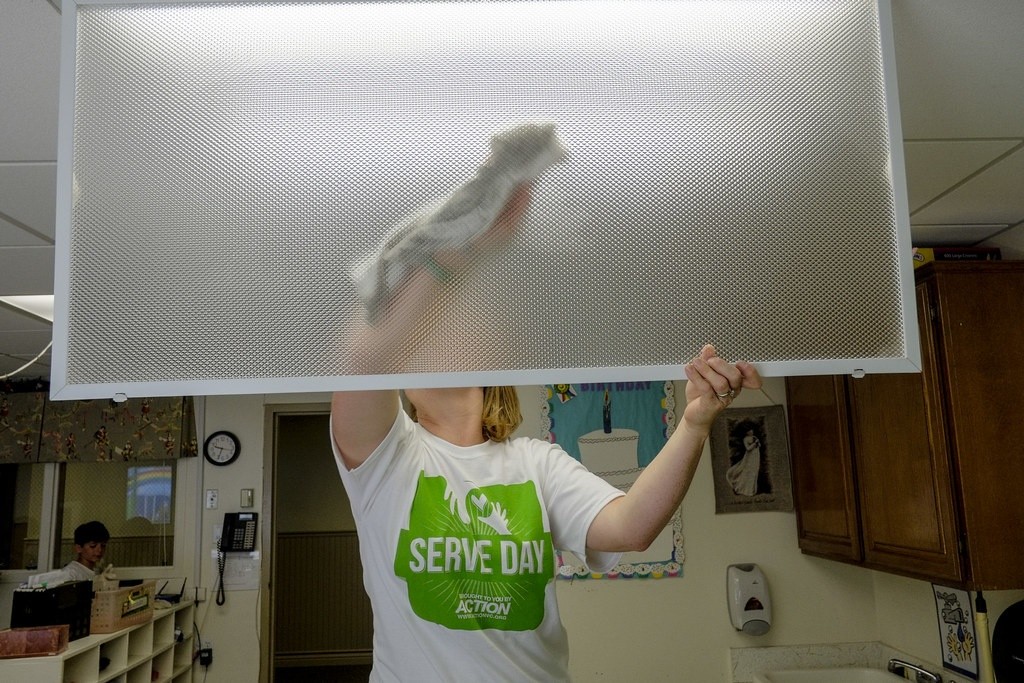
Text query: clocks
203 430 241 466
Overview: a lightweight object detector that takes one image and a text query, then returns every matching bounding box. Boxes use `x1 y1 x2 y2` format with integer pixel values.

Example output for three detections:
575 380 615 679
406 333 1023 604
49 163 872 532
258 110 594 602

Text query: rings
718 389 736 397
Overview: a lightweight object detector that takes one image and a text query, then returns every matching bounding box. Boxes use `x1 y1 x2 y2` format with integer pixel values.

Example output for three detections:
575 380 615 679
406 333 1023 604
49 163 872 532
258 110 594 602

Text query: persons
329 185 763 683
725 427 762 496
57 520 111 582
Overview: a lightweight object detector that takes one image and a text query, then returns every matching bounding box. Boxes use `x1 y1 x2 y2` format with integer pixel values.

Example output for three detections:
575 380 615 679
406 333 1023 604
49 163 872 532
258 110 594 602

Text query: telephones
219 511 259 553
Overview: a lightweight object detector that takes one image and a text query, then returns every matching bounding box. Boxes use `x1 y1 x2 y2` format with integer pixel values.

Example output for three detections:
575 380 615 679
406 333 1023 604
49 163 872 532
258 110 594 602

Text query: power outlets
201 638 216 658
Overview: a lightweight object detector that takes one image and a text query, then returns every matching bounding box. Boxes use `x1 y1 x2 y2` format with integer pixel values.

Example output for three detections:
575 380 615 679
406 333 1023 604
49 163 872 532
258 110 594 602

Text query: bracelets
424 257 456 285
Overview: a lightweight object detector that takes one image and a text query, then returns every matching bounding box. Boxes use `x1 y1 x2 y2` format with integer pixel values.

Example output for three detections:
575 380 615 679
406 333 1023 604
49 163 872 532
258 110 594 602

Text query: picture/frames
930 583 980 683
539 380 686 581
708 404 795 515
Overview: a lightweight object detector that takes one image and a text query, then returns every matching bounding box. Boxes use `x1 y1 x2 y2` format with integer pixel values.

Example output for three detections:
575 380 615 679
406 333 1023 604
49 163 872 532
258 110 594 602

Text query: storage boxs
0 624 70 658
90 578 159 634
10 579 93 643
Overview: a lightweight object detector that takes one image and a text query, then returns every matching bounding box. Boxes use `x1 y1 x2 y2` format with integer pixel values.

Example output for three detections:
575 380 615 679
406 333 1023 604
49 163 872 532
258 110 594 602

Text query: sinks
764 667 915 683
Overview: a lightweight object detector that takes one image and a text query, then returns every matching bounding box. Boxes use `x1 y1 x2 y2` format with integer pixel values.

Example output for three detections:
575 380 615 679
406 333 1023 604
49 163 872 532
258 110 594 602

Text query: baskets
90 578 156 634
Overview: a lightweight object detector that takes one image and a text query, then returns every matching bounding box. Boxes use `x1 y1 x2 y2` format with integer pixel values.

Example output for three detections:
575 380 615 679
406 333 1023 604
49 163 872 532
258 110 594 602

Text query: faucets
887 658 940 683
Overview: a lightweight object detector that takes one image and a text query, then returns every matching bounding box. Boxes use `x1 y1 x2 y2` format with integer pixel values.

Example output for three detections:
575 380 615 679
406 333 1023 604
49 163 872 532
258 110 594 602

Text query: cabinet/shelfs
0 599 196 683
787 257 1024 593
